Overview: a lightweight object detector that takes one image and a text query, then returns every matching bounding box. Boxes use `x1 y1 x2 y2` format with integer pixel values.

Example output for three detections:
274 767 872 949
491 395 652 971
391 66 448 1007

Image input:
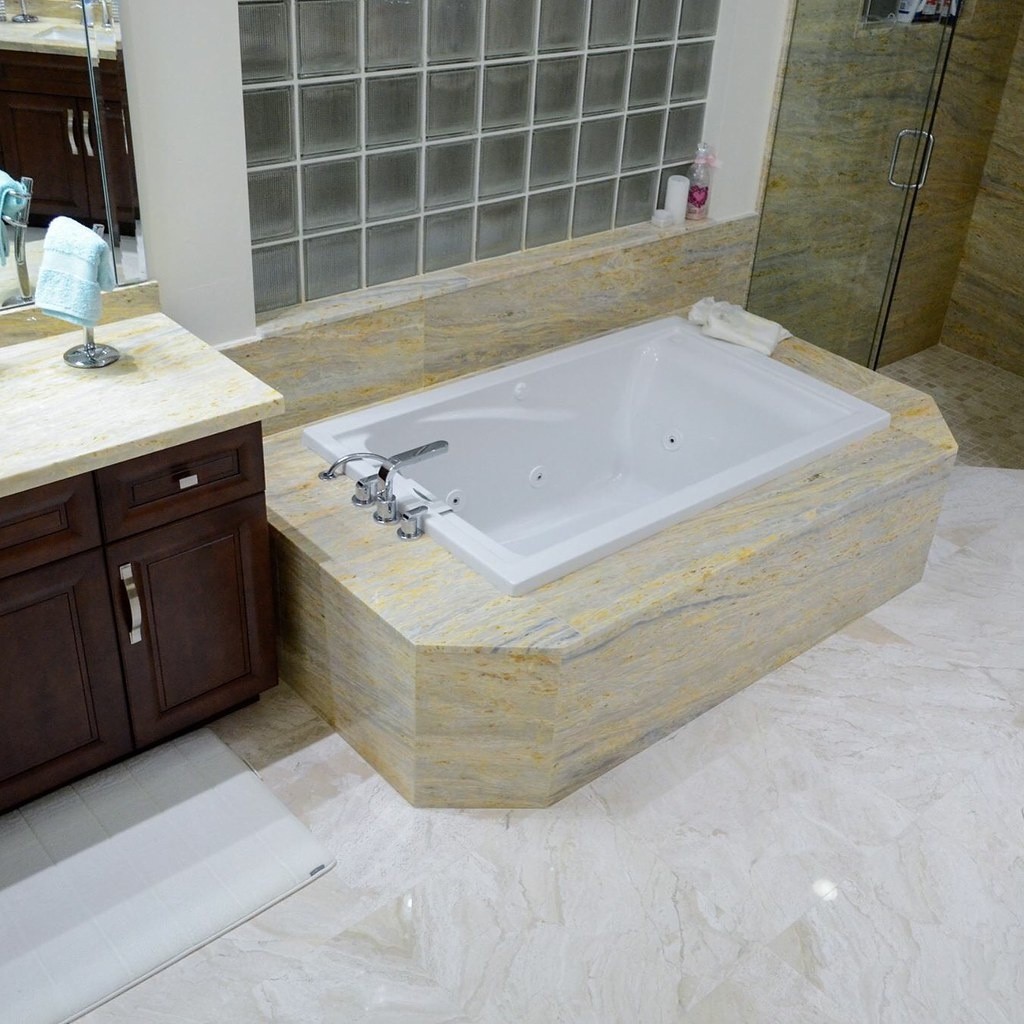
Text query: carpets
0 726 337 1024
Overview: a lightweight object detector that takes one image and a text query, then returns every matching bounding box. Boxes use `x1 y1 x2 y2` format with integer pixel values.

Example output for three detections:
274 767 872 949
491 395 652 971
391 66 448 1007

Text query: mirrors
0 0 148 313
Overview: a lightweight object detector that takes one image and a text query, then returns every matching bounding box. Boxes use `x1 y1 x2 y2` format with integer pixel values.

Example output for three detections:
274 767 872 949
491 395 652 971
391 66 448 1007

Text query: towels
0 169 28 267
34 216 117 329
688 295 794 357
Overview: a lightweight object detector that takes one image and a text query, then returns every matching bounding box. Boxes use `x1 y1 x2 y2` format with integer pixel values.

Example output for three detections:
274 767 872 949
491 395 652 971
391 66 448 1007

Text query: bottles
686 142 711 220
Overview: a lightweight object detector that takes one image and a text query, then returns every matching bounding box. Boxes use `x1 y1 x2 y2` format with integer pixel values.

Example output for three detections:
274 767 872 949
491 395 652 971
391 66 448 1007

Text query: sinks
33 25 115 45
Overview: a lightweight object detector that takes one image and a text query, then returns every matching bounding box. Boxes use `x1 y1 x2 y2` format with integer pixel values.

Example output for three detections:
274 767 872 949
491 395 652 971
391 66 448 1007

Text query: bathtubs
263 303 959 811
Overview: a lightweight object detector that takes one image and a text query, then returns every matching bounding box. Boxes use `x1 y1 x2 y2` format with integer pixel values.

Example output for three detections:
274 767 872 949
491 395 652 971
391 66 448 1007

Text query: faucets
69 1 85 27
372 440 449 526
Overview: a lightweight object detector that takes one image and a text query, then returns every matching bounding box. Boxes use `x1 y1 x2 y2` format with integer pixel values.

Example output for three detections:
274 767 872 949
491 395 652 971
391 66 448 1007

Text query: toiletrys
895 0 959 26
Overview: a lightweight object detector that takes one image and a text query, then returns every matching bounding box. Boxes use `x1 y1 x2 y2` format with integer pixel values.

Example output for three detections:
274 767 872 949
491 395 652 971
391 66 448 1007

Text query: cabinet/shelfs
0 420 279 819
1 50 141 237
